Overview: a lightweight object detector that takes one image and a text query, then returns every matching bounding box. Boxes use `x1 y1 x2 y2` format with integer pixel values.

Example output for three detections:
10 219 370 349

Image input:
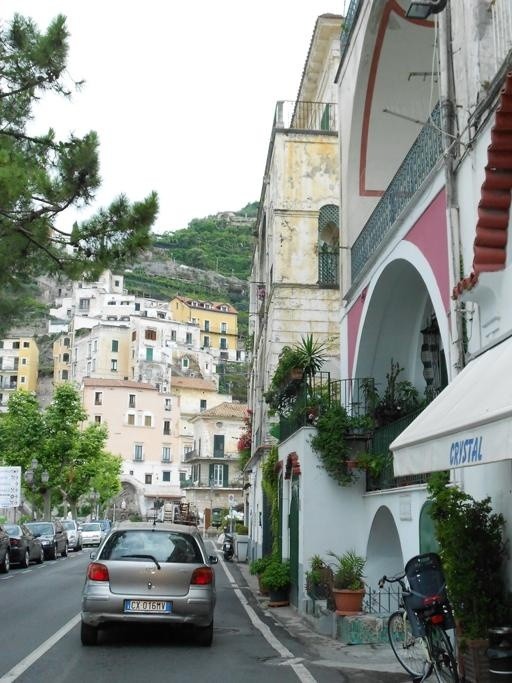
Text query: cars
0 514 118 577
79 519 222 648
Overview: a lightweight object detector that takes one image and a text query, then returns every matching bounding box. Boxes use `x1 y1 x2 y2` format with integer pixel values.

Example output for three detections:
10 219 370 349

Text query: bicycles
371 562 473 677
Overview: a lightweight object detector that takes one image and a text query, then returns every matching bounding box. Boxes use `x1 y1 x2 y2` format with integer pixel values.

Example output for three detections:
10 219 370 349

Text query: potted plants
262 345 306 411
249 556 291 607
426 470 512 683
325 548 366 612
